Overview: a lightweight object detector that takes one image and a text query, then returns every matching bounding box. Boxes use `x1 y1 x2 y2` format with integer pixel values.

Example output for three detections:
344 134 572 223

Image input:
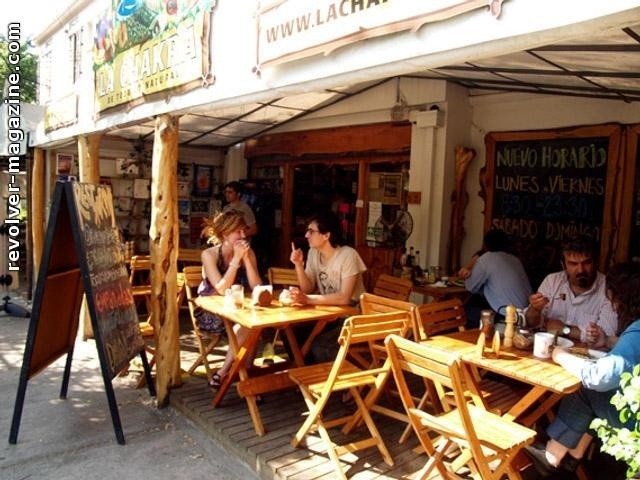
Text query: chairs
384 333 537 480
360 292 446 445
410 297 529 462
182 265 227 381
342 274 413 404
287 310 411 480
267 267 298 349
119 272 184 390
128 255 156 296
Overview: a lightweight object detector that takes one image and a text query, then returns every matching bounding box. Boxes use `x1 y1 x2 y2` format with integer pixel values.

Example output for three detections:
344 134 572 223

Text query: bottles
480 311 494 343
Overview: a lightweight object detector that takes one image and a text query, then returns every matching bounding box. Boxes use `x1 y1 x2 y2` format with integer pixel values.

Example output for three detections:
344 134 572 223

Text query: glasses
305 227 319 234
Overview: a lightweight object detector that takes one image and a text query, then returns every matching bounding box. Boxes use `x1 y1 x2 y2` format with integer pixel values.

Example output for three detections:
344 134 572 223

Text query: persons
524 241 617 345
288 212 368 365
223 182 257 241
523 259 640 479
194 209 263 387
457 250 485 277
462 229 534 329
587 320 624 349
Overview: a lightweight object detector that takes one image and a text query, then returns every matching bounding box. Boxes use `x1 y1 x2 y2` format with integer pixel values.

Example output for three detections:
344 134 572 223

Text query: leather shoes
522 444 558 479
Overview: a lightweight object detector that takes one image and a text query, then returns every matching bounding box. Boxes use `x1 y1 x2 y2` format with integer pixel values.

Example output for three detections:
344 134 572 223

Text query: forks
544 295 564 301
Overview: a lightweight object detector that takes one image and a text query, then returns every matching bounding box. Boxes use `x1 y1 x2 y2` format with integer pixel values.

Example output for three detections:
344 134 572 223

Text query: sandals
207 372 222 389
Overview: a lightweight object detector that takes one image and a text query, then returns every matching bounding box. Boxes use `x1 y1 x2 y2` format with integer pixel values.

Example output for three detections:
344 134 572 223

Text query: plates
555 335 573 349
453 278 465 287
564 347 609 359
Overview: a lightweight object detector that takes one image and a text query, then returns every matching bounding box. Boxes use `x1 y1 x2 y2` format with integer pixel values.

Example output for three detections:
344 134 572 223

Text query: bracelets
229 263 241 268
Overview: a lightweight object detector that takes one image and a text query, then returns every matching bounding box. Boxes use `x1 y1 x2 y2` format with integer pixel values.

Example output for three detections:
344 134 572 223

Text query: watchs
295 262 305 268
562 324 572 337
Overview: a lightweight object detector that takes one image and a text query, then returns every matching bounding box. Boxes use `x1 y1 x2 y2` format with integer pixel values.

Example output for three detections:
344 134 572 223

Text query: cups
232 284 245 310
441 277 448 286
533 333 555 359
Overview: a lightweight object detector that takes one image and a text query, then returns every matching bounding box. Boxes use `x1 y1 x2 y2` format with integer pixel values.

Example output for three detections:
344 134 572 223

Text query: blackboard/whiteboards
65 184 146 382
484 125 624 277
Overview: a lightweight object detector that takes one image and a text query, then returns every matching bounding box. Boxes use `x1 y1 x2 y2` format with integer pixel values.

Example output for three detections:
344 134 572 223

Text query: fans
373 208 414 248
